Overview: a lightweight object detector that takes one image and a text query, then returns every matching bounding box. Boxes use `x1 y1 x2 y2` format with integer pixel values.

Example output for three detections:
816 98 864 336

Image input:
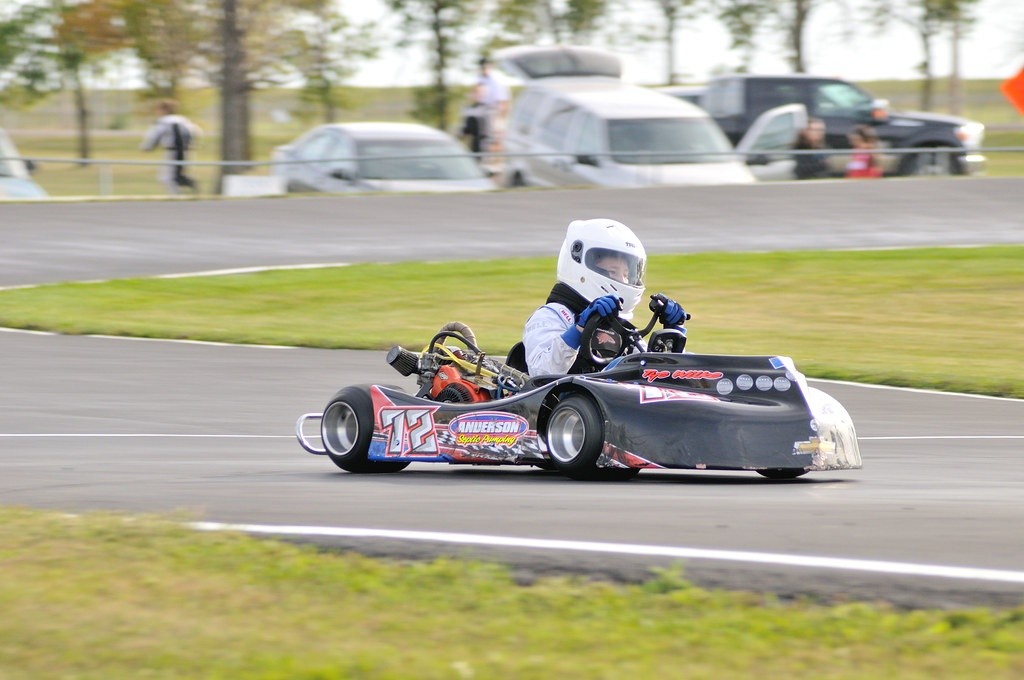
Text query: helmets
556 218 647 320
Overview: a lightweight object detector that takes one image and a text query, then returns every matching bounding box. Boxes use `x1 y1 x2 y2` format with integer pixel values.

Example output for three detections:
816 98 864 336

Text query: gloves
659 298 687 329
576 294 624 330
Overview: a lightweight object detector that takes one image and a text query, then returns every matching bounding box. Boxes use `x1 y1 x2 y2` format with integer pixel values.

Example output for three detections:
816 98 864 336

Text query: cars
0 127 48 198
267 120 500 194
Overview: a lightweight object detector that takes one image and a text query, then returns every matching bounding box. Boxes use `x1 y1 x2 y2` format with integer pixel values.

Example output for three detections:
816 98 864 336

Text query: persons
793 118 834 179
142 102 198 193
521 218 687 375
456 57 511 162
845 126 885 179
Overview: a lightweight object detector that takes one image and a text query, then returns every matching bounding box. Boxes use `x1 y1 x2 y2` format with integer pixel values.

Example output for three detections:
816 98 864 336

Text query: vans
482 44 808 190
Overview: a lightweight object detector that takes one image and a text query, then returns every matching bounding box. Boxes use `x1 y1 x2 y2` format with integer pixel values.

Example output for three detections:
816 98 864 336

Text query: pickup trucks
699 71 987 180
